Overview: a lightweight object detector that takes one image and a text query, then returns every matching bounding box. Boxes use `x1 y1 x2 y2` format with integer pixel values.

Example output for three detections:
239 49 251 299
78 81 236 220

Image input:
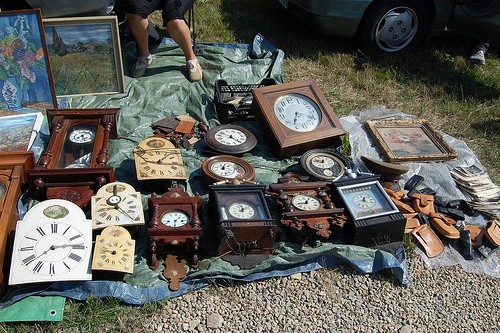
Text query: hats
361 156 410 181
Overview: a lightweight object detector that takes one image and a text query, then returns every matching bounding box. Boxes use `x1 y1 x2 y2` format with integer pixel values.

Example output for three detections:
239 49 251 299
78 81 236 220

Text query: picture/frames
0 112 44 151
0 8 58 116
365 119 458 164
42 16 126 98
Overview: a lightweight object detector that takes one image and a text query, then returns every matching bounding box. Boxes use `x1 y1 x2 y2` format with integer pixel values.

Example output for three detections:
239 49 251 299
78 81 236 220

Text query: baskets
214 78 277 124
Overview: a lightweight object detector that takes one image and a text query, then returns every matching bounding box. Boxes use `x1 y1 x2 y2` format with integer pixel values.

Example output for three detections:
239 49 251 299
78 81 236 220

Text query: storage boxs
215 78 279 125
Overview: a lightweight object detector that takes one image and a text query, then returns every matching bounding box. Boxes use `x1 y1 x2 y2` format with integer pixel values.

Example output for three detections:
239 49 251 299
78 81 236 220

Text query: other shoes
131 53 153 78
186 57 202 81
468 49 485 65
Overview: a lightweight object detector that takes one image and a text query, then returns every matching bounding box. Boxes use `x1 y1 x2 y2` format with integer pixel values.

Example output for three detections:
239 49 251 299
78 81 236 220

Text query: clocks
0 79 407 300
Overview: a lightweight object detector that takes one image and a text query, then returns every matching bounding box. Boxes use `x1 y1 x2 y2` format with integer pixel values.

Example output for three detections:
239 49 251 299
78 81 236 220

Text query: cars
277 0 500 65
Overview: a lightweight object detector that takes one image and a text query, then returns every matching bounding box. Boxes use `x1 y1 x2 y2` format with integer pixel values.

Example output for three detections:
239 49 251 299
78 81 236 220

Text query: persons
470 43 491 65
127 0 203 82
